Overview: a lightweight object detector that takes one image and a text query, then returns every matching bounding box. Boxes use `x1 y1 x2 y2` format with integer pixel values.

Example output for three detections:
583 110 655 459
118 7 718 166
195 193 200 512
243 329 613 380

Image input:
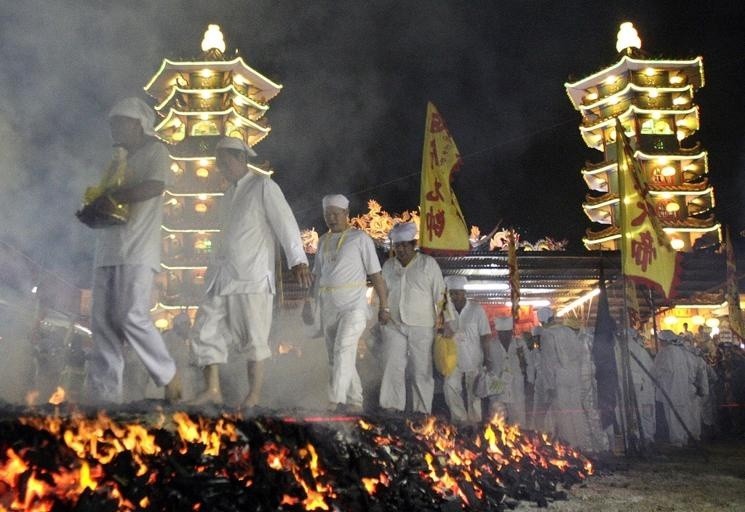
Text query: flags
722 230 745 341
419 100 476 260
613 120 686 304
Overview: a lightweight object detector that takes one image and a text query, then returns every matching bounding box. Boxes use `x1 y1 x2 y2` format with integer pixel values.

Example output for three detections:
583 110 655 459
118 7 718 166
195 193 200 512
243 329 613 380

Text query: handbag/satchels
430 331 458 378
473 364 506 399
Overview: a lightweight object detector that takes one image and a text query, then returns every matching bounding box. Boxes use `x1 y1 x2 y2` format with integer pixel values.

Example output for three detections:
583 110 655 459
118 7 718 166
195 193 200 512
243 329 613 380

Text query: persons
163 314 195 404
1 232 38 409
442 275 718 454
372 219 458 410
80 94 189 404
294 191 394 418
187 137 313 409
